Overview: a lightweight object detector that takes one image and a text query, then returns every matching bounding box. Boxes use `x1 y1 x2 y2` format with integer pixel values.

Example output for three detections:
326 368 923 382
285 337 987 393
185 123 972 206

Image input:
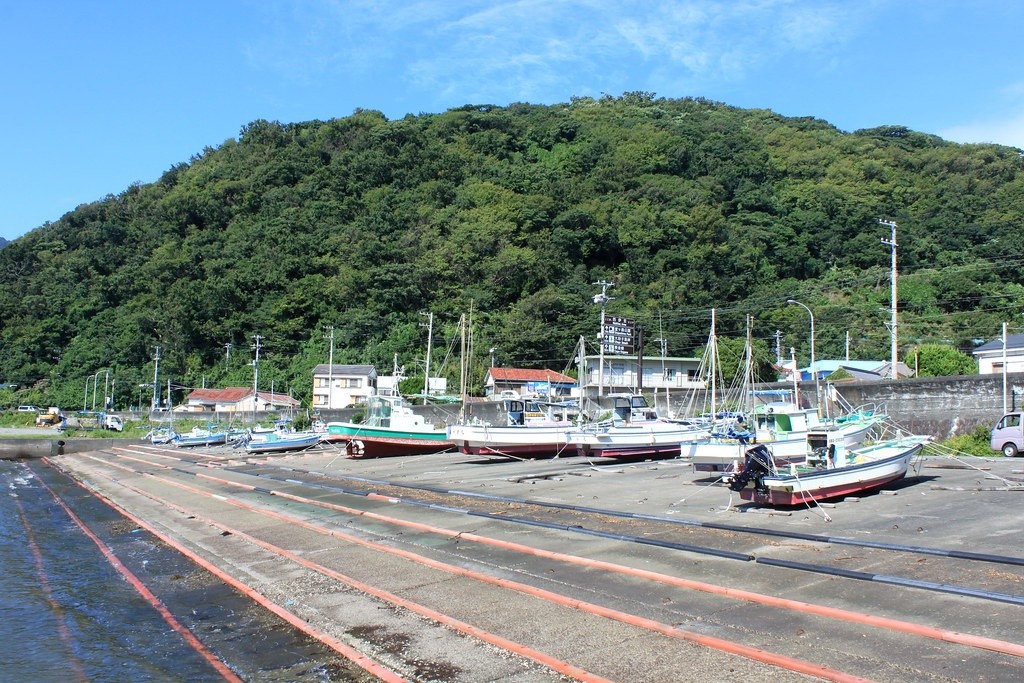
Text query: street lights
653 339 668 357
84 370 109 411
490 348 497 367
788 300 815 380
139 346 162 412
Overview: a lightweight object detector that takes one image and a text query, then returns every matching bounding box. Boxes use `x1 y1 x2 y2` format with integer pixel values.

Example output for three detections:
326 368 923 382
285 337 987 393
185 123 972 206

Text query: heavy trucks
66 415 124 433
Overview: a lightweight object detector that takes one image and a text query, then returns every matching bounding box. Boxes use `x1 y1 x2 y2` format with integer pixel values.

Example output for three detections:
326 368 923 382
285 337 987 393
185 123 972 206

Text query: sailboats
140 308 890 474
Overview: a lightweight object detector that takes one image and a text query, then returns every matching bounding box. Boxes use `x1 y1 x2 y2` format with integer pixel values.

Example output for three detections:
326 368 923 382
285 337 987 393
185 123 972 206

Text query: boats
722 379 924 505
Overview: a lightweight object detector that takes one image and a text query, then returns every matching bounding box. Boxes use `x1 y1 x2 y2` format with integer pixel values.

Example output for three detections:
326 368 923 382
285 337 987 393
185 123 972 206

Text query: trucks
990 412 1024 457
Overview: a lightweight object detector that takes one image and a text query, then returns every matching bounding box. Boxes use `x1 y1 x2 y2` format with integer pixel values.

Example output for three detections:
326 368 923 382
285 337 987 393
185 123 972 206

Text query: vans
18 406 35 411
